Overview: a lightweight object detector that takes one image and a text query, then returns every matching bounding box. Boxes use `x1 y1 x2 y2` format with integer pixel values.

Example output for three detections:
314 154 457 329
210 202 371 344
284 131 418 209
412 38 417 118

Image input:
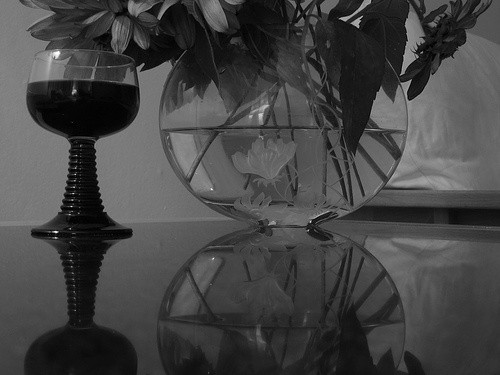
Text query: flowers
16 0 493 207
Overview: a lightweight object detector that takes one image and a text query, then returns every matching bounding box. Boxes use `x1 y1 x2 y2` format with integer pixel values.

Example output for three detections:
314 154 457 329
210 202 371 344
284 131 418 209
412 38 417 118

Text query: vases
156 18 409 228
152 225 408 375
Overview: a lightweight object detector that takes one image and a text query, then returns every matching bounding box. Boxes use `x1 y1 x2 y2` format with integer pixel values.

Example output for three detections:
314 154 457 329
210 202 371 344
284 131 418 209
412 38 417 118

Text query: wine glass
26 46 141 239
24 234 138 374
